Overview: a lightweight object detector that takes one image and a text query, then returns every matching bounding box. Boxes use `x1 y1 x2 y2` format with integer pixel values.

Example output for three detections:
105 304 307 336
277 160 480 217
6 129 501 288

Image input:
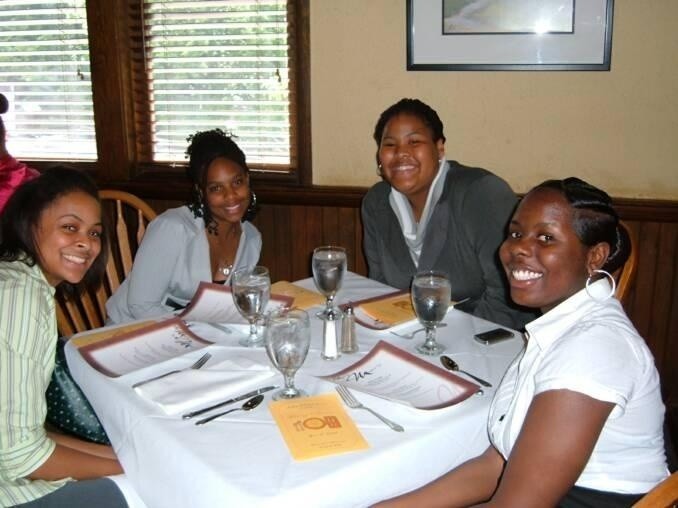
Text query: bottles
340 306 358 353
320 312 340 362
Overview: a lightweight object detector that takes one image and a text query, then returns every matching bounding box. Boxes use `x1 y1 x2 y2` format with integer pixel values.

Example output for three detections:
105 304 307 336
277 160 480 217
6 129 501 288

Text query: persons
2 168 127 508
368 176 672 508
356 98 542 335
0 95 47 217
101 129 266 325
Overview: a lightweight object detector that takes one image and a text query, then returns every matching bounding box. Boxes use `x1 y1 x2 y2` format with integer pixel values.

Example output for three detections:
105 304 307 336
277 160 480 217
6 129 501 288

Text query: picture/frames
405 1 614 71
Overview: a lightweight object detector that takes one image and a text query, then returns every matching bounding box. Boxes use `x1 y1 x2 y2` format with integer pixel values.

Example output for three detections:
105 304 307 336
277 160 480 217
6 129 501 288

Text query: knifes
182 384 278 421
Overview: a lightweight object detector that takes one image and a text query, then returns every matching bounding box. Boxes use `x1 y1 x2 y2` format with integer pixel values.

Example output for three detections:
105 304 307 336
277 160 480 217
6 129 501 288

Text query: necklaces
216 264 236 278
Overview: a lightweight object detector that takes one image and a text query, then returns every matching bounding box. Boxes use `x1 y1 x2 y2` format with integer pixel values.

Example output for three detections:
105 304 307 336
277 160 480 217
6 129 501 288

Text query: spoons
440 355 493 390
194 393 264 427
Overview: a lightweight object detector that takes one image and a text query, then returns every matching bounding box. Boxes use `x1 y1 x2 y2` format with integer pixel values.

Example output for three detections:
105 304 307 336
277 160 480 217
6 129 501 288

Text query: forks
132 352 211 390
389 323 449 339
334 383 405 434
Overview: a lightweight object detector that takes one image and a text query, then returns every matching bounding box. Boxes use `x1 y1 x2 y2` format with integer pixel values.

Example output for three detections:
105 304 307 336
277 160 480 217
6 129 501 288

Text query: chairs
50 188 159 343
609 219 639 303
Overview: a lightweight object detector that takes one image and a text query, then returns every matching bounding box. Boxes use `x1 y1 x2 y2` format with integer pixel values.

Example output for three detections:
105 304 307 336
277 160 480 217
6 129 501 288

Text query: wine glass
231 265 272 348
311 245 349 319
264 307 311 401
411 270 451 358
